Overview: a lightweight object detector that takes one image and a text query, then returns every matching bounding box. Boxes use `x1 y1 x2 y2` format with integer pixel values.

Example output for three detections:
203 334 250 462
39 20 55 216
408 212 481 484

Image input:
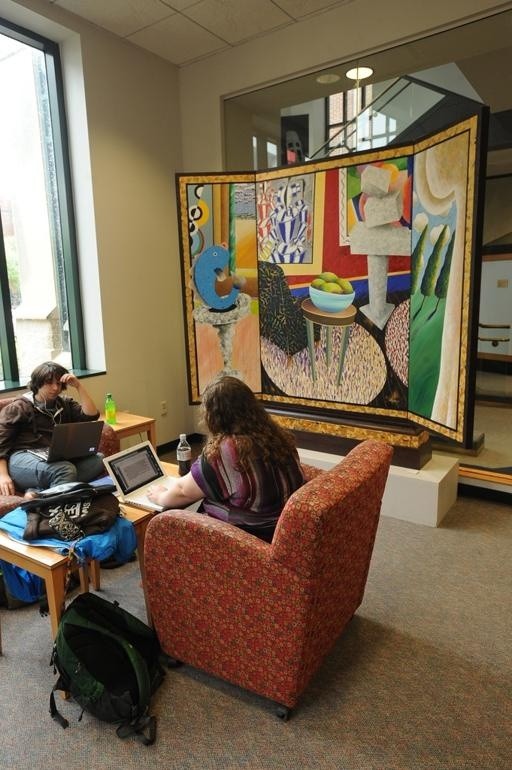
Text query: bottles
177 433 193 476
104 394 117 425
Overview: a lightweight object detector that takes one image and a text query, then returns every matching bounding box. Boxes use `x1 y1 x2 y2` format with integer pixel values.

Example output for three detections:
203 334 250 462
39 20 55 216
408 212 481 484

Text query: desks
1 461 199 646
94 409 158 462
299 298 359 388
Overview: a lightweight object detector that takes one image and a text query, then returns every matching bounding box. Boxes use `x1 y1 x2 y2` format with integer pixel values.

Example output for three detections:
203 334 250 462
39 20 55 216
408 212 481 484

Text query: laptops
102 440 185 513
25 420 104 463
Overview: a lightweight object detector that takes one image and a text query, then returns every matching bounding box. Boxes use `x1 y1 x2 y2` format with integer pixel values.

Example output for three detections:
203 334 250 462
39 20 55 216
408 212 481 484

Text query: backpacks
47 592 166 746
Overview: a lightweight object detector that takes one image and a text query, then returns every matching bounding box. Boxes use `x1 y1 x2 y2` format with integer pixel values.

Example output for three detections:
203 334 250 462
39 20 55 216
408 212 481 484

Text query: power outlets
160 399 168 416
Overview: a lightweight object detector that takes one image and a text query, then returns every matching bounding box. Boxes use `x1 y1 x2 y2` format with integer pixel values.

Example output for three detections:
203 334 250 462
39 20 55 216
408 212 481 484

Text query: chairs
143 436 396 722
256 258 321 369
1 396 121 518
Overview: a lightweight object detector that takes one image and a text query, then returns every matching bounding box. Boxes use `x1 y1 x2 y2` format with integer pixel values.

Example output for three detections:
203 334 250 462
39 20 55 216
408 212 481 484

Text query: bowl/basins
309 286 355 313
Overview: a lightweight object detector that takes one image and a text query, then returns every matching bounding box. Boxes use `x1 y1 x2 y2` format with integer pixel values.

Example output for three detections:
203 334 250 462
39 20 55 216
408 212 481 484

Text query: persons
0 360 99 499
147 376 305 542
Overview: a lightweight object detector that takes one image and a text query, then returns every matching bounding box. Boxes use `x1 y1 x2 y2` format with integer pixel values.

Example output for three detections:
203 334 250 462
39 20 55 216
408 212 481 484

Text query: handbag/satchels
23 482 121 540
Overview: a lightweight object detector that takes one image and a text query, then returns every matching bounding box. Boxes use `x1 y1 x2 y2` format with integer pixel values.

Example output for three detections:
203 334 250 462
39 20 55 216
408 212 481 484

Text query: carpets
262 321 388 406
385 297 409 391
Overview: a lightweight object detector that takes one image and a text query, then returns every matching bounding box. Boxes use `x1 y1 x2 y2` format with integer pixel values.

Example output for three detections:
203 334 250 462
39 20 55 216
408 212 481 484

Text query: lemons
310 272 354 295
